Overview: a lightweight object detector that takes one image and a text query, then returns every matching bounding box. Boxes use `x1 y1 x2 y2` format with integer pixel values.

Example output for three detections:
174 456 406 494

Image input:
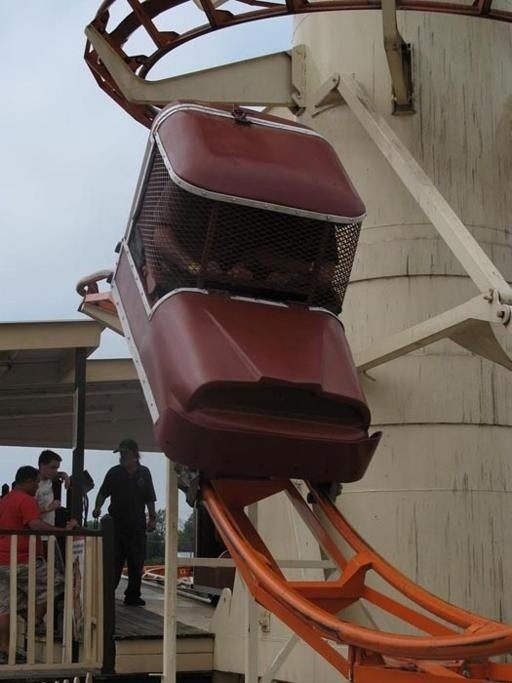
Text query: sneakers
0 649 27 663
24 622 60 642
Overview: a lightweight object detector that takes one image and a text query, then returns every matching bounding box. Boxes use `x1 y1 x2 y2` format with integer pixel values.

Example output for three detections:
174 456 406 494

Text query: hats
113 438 139 454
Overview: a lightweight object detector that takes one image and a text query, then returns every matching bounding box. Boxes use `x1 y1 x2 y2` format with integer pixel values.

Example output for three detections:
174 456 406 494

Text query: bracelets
43 503 51 512
308 260 315 271
186 261 203 276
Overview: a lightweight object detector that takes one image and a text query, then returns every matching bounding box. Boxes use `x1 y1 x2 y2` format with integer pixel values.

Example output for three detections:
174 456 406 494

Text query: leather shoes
125 595 146 606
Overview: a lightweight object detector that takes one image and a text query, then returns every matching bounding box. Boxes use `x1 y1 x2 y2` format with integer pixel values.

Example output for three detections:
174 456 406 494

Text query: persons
92 438 158 606
32 449 71 616
2 465 79 665
144 176 255 301
264 211 343 317
